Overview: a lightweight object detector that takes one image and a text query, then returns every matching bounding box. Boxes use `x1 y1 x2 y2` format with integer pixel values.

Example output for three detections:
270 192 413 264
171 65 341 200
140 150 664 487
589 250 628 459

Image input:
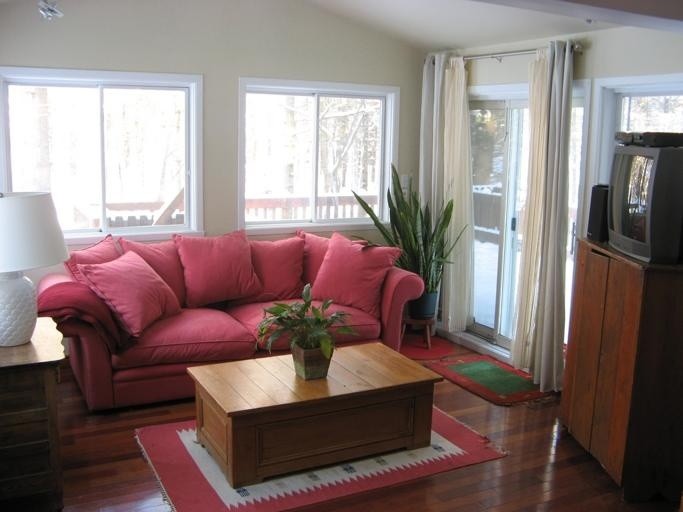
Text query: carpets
400 335 454 360
423 355 556 406
135 405 509 511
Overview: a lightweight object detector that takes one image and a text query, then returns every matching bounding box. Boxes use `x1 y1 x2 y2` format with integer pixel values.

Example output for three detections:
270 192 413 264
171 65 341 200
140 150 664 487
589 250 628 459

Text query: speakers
584 184 609 243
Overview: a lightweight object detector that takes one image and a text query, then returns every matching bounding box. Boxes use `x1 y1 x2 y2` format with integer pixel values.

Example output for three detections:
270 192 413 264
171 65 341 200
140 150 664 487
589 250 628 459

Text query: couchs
36 266 425 413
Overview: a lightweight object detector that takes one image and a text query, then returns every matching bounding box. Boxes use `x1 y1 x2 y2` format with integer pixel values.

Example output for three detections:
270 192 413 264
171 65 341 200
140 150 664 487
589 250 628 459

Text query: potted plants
254 283 362 380
352 163 469 321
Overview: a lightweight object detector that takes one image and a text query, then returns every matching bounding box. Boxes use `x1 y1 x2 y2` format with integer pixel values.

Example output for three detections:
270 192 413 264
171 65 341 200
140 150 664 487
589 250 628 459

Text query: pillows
227 234 305 306
297 228 368 287
172 229 262 309
64 234 121 284
76 250 182 338
311 230 403 319
117 237 186 309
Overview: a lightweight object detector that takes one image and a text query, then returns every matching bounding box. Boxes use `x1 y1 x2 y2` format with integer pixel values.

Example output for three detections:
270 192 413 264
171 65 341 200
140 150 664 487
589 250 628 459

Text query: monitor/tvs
607 145 683 266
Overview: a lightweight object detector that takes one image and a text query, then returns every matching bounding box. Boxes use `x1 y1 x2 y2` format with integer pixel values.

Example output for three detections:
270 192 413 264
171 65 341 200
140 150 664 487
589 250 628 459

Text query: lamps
1 192 71 347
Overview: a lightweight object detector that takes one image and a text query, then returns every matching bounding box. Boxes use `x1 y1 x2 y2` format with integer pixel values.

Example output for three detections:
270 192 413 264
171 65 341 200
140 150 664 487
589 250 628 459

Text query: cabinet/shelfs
560 235 683 504
0 317 66 512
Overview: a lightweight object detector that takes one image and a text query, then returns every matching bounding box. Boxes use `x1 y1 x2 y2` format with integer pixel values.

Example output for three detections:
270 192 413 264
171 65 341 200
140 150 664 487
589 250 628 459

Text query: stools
401 318 435 350
